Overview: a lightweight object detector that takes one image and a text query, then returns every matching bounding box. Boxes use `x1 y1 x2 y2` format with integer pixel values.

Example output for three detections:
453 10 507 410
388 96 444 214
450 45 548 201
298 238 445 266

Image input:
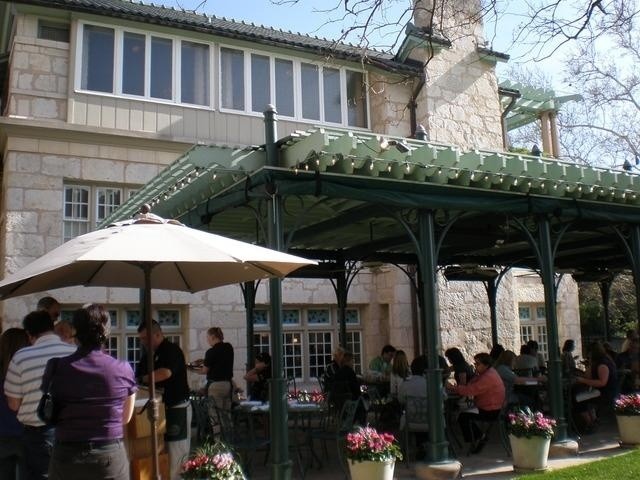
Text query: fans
326 222 413 278
440 248 504 278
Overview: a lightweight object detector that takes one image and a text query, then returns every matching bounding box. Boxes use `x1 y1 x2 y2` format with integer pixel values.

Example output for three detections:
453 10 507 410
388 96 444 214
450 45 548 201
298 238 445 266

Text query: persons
1 296 139 478
134 318 195 479
325 331 638 456
181 327 233 450
243 352 274 401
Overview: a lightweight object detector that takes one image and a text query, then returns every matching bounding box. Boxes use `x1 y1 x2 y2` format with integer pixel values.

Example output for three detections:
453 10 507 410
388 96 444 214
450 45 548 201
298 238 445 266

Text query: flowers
181 438 244 480
614 389 639 413
342 421 402 463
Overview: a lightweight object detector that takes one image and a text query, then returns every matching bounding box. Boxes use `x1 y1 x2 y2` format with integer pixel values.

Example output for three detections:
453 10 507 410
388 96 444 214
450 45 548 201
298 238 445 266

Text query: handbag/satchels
37 392 56 427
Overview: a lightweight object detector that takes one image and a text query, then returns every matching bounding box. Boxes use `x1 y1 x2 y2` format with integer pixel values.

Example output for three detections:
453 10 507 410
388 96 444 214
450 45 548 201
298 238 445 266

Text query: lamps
374 134 413 158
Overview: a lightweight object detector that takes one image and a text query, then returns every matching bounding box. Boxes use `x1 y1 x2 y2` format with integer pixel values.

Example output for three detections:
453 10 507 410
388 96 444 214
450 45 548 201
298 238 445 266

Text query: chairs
186 383 337 459
344 358 601 447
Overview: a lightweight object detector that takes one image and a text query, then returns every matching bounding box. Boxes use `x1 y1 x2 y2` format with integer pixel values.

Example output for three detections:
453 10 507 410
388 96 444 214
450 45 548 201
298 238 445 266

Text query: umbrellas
0 206 320 478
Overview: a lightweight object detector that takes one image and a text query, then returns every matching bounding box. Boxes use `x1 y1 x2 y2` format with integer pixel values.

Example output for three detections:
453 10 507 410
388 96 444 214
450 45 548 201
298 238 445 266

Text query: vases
347 454 397 480
507 430 555 471
615 408 638 445
